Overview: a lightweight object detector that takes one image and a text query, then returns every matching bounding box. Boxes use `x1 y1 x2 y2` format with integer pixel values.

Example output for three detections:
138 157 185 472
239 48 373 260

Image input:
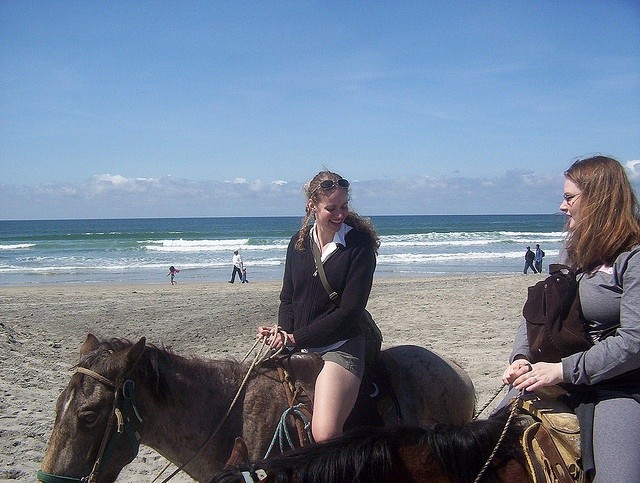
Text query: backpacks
523 264 595 362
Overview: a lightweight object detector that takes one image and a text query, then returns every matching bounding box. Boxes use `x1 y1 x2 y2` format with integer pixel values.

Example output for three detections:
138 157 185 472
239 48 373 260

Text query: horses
34 331 478 482
212 405 591 482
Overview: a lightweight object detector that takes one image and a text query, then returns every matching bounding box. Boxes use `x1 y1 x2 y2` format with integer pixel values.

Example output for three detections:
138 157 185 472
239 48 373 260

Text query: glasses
564 193 581 203
309 180 350 197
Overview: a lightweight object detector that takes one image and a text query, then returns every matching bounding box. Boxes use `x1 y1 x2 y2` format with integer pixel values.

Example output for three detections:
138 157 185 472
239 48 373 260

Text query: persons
524 245 538 275
167 265 180 285
228 249 243 283
486 154 640 483
241 266 249 283
254 170 383 444
534 243 543 274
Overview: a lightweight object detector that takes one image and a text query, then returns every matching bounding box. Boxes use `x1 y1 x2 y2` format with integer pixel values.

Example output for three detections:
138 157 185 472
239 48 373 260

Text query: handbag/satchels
361 310 381 368
